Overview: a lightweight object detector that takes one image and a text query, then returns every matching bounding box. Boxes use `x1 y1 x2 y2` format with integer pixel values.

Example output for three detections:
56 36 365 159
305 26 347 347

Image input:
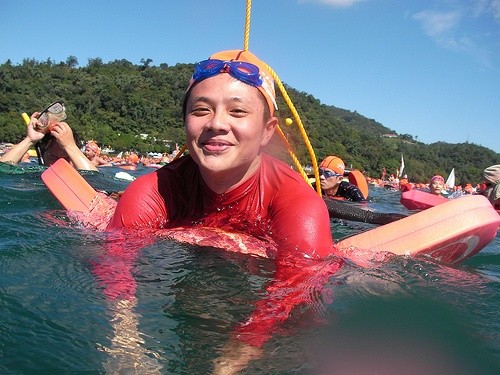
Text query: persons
0 102 500 209
88 48 345 375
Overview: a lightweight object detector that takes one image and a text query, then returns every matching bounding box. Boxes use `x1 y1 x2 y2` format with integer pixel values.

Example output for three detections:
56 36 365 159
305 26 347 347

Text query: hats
129 154 138 163
466 184 472 189
480 184 487 191
184 50 276 118
87 142 98 153
318 155 345 176
400 179 408 185
430 175 444 185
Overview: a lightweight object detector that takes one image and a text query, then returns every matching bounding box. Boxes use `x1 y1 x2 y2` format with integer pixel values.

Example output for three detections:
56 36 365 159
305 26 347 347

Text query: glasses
34 101 66 133
319 169 342 178
193 59 262 86
